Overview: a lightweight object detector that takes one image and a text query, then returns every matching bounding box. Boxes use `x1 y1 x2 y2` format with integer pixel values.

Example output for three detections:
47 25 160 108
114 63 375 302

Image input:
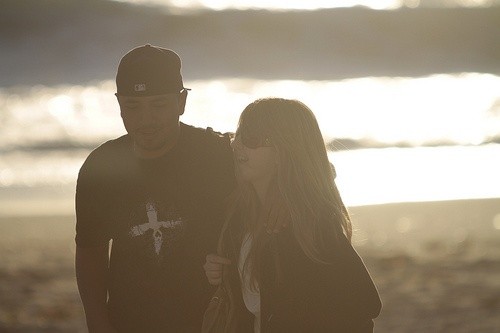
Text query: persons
75 44 336 333
204 98 382 333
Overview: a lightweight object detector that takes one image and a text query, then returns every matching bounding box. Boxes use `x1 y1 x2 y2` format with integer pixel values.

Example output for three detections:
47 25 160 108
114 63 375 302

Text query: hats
115 44 191 97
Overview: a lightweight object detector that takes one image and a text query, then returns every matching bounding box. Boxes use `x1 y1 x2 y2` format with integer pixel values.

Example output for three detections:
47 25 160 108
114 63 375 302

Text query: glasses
234 128 278 149
